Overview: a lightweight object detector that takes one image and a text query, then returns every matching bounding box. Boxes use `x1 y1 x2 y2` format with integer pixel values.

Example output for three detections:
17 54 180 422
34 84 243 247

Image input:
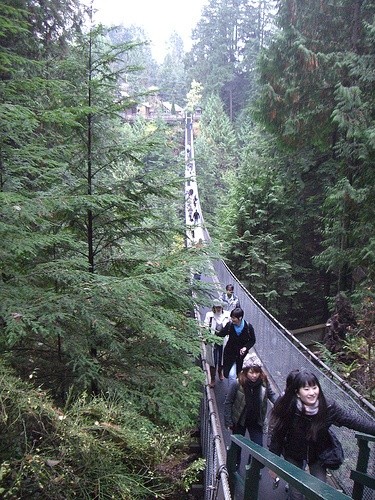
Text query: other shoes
218 371 225 383
245 464 262 479
285 482 290 492
209 379 216 388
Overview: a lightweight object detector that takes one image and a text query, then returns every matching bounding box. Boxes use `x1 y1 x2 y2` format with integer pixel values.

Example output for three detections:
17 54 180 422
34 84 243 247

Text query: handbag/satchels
319 429 345 470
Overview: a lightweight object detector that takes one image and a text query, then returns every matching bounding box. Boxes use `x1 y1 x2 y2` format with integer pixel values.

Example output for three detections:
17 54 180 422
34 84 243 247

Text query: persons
193 272 201 280
215 308 256 379
225 354 276 480
203 299 231 387
186 142 201 237
269 372 375 500
267 369 303 447
218 284 240 312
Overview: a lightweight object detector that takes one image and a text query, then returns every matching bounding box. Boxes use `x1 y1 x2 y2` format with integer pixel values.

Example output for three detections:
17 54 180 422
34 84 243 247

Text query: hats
212 299 224 308
242 352 264 368
